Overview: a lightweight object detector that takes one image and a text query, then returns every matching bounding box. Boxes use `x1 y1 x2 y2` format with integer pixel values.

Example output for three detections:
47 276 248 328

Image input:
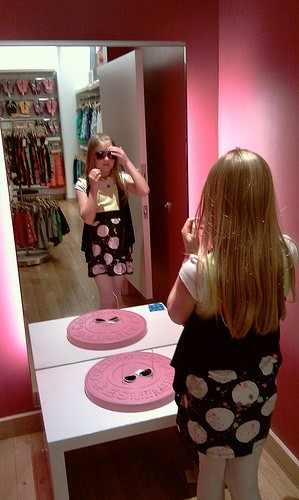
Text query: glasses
122 348 154 383
92 292 120 324
95 151 116 161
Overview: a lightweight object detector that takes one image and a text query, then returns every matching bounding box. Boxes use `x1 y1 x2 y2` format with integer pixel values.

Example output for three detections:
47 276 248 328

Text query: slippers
0 79 59 133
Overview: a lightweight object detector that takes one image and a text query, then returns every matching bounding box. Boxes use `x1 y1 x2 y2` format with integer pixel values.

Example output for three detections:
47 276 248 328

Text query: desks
26 301 184 370
36 344 217 499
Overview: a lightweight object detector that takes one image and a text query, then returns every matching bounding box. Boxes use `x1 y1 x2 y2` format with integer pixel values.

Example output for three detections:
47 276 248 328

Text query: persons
166 148 299 500
74 133 150 310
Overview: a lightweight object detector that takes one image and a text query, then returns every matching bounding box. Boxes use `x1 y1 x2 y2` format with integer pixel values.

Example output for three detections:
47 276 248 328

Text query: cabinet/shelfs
76 79 102 183
0 68 67 202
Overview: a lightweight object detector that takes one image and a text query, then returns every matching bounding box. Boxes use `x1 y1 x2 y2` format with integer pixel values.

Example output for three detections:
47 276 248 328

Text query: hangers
12 198 63 212
2 126 51 138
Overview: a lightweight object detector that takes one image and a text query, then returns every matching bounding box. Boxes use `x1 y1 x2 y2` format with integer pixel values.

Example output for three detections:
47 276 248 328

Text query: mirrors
0 40 188 368
